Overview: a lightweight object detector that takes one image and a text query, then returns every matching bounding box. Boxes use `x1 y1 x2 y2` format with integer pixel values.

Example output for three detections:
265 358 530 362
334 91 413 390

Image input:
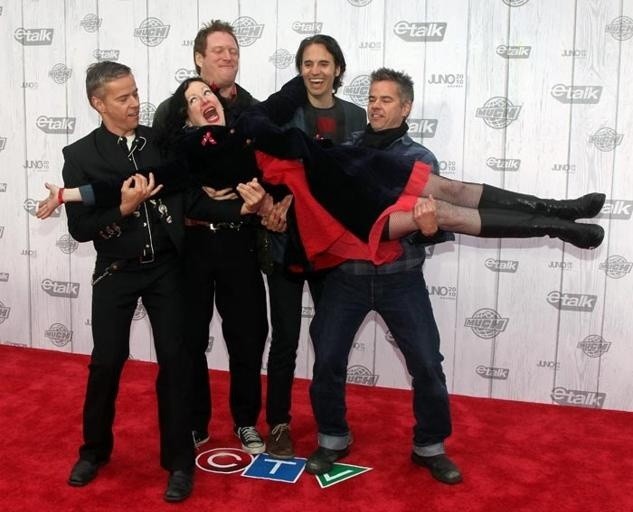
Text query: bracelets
58 188 64 203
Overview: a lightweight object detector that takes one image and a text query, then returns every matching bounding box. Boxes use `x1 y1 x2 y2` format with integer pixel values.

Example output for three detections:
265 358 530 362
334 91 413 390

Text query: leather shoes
164 460 193 503
68 449 110 487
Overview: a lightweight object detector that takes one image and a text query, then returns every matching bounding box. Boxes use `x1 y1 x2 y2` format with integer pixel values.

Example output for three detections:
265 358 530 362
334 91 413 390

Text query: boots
476 207 606 250
477 182 607 221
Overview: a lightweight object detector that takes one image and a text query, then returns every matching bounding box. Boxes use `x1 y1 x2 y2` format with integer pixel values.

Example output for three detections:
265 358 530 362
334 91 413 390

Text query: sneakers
265 422 296 460
189 428 210 449
411 447 462 485
305 443 349 475
233 422 266 456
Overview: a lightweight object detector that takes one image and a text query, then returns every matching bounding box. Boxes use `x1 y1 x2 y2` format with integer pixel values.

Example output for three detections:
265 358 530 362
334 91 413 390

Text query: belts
184 218 240 230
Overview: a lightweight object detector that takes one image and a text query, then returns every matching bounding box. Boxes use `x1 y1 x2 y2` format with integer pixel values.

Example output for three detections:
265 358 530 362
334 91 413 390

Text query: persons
303 67 464 486
264 34 368 461
61 59 266 502
150 18 270 457
36 72 607 250
